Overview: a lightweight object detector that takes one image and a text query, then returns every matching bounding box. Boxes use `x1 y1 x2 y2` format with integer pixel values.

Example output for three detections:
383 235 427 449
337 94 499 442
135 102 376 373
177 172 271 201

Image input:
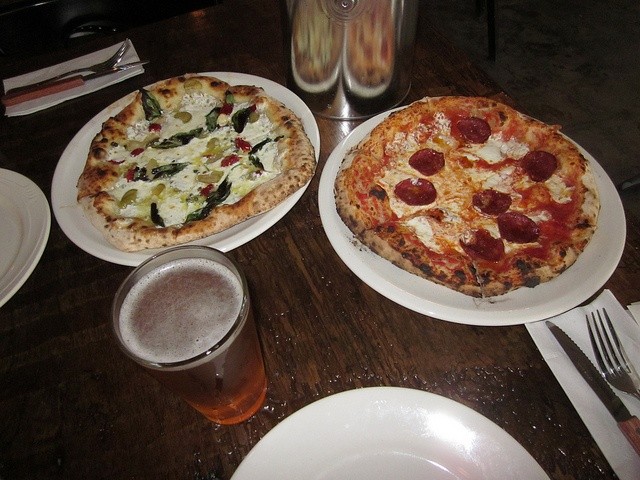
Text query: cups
111 246 267 424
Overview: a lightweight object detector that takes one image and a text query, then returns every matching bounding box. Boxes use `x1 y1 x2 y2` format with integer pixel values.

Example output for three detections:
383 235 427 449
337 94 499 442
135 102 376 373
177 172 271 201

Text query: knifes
2 58 153 105
545 320 640 462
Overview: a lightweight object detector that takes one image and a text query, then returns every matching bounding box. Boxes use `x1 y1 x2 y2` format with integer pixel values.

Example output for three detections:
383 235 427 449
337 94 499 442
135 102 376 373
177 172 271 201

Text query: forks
583 307 640 405
7 38 132 100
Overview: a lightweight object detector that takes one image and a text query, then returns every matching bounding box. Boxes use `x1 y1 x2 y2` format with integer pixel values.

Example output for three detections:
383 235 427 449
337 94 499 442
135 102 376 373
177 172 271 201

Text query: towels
524 288 640 480
2 40 146 116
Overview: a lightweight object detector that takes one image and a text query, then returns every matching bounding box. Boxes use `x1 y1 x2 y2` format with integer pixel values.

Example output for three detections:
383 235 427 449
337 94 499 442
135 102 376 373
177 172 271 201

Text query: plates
341 50 396 99
51 70 320 268
291 41 343 93
230 387 556 479
317 98 628 329
0 164 51 317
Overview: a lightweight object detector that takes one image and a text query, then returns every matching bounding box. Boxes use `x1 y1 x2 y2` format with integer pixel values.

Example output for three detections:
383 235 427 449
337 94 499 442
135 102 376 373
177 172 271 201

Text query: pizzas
334 94 599 298
78 72 316 250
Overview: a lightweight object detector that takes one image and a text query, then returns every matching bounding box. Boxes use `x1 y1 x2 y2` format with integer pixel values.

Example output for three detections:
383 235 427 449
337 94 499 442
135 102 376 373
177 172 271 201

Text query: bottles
281 1 420 120
